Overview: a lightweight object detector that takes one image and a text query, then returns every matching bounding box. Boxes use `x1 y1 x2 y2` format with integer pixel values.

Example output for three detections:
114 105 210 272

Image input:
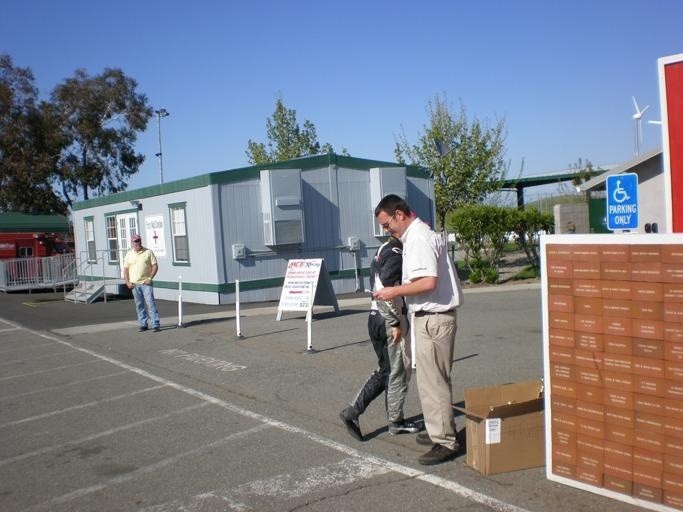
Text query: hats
131 235 141 242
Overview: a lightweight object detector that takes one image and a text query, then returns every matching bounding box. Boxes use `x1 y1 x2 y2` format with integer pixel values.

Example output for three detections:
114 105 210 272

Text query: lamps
129 199 142 210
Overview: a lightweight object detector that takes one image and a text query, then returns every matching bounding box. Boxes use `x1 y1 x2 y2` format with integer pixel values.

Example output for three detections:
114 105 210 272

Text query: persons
123 234 160 332
340 236 423 441
372 195 463 465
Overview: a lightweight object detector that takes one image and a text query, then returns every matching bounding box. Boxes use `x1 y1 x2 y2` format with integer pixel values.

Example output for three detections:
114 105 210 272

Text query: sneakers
151 326 160 332
415 433 433 446
338 405 364 442
418 442 464 466
388 418 424 436
136 326 148 332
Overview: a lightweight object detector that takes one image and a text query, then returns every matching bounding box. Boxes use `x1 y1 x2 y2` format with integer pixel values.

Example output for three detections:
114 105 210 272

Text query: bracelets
148 277 152 281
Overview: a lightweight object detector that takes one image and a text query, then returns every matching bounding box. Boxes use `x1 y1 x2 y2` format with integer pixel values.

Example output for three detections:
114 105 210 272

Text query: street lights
148 105 169 184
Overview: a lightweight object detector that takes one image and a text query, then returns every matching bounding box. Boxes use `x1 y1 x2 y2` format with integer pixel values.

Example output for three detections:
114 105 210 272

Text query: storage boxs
448 379 545 478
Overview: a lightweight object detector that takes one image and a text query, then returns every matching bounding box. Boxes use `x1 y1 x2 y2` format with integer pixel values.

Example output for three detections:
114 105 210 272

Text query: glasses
380 213 398 229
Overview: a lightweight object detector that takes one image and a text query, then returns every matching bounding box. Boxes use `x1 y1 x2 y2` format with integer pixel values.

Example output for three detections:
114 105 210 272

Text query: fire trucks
0 231 75 286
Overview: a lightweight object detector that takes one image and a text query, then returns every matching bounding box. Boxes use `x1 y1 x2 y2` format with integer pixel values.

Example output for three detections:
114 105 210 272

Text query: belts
414 309 454 317
